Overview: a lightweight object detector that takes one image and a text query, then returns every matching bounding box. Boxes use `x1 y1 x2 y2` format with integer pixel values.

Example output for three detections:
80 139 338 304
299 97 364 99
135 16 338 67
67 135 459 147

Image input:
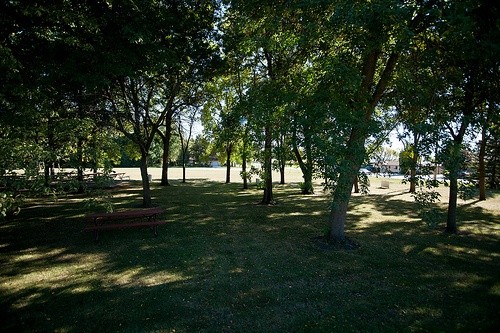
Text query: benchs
82 208 167 240
